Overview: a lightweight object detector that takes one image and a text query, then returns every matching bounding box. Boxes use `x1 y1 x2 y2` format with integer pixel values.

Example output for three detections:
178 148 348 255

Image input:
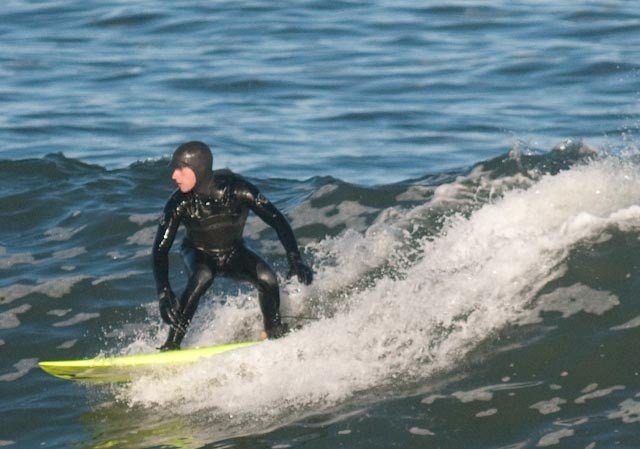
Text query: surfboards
39 341 279 382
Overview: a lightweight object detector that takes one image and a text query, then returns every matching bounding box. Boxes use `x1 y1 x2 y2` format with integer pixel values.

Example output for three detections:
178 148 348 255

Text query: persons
151 140 313 352
